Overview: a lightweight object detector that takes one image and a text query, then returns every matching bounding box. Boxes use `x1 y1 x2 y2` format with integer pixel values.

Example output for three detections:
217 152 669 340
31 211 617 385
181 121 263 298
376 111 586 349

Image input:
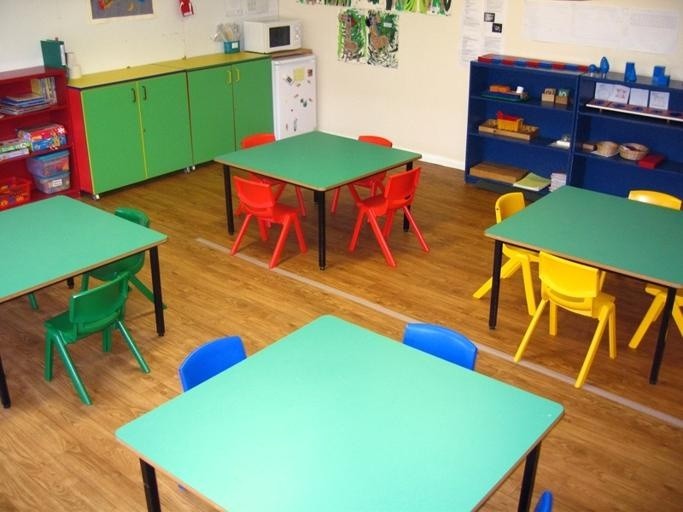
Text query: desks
483 185 682 387
206 129 422 270
0 194 169 409
113 315 564 510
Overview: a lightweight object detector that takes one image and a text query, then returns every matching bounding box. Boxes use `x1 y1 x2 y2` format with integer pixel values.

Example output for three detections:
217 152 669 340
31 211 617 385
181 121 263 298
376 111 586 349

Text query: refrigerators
271 57 316 140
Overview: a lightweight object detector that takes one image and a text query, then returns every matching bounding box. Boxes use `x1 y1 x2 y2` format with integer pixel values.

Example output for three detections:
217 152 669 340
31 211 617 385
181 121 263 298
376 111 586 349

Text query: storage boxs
26 150 71 179
31 172 70 195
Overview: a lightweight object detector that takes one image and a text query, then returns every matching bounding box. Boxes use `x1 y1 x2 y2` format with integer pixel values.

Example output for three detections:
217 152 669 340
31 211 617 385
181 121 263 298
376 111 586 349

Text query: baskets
620 143 649 160
597 141 618 153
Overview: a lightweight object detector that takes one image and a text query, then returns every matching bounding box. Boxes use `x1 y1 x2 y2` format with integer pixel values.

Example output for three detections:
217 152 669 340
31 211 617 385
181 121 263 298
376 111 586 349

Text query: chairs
176 335 247 388
626 189 683 212
77 206 169 328
41 272 147 406
511 251 619 389
401 320 476 373
229 176 306 266
346 166 429 270
236 134 307 221
533 489 553 510
625 280 682 350
471 191 544 315
328 135 392 215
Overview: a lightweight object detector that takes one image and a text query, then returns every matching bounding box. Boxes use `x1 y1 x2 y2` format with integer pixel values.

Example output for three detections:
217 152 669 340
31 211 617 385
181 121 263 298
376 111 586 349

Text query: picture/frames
86 0 155 23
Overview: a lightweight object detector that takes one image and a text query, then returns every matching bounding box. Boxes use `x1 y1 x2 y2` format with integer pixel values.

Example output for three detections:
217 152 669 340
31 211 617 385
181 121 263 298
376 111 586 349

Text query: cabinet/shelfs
151 51 275 173
0 66 83 211
567 68 682 209
462 59 587 200
65 63 192 202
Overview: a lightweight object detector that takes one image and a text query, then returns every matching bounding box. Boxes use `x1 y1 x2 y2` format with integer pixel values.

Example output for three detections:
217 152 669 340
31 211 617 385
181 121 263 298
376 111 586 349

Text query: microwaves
242 15 301 54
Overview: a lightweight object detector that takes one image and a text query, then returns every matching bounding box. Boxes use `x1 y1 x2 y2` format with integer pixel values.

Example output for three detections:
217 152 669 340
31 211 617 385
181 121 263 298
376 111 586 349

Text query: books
513 171 550 192
1 76 58 115
